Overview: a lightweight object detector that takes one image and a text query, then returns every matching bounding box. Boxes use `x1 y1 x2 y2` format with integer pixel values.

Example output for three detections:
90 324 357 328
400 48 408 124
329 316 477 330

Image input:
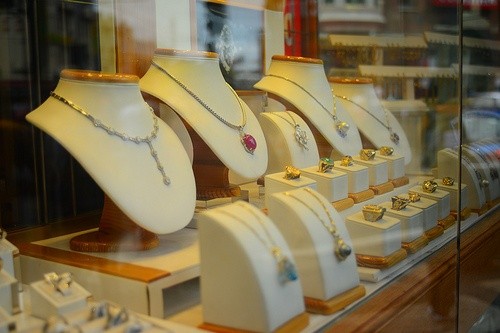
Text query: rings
57 274 72 291
360 149 376 160
341 154 353 166
380 145 394 155
392 196 409 209
423 180 437 193
284 166 301 180
362 204 385 222
319 157 333 173
409 192 420 203
97 301 144 333
443 177 455 186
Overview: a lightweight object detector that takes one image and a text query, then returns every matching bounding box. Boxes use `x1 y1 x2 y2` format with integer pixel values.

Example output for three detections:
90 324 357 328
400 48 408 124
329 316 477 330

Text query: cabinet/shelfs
15 89 500 333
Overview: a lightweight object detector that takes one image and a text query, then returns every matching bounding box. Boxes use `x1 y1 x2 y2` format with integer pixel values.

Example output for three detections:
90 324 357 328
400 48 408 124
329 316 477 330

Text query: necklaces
451 138 500 189
51 92 170 185
271 111 310 152
152 61 256 155
265 72 349 136
338 95 400 143
285 189 352 261
222 205 298 282
